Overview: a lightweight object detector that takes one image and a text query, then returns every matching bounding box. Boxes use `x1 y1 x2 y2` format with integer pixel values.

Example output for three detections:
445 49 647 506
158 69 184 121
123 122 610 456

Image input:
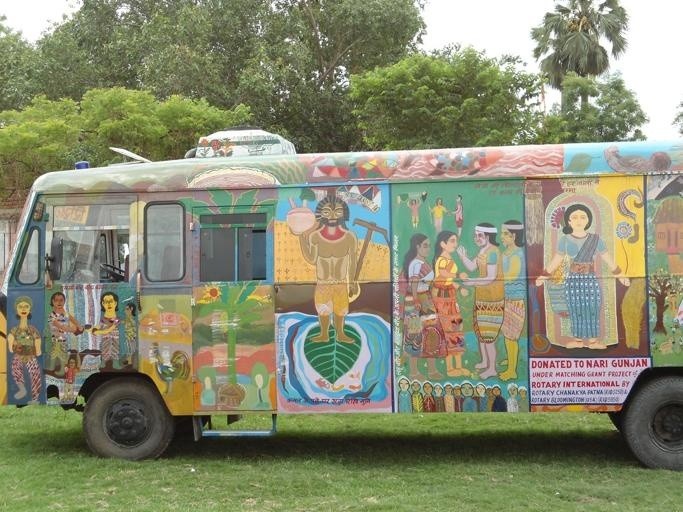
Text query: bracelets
540 267 551 279
612 266 620 276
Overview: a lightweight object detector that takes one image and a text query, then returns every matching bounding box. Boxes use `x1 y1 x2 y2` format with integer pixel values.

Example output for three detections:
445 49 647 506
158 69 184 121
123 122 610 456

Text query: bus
0 129 683 473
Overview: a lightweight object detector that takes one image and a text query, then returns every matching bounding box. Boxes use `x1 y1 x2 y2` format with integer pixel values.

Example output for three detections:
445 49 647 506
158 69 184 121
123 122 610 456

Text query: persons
533 202 635 350
7 291 141 408
288 195 363 344
398 189 530 386
